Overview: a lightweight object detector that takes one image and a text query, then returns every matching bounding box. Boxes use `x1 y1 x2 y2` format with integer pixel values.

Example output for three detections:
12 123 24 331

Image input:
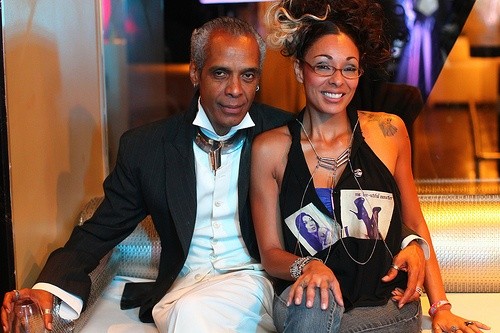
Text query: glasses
299 58 365 80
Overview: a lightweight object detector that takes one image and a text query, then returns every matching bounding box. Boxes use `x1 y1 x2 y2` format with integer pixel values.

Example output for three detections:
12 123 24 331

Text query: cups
13 299 46 333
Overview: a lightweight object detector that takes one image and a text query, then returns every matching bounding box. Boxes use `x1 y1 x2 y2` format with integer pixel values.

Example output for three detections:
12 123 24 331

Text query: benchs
19 177 500 333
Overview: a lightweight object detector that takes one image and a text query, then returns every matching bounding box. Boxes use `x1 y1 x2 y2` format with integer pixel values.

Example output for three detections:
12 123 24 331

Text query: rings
43 309 52 315
298 283 304 289
450 325 459 332
465 321 473 327
13 290 19 299
416 287 423 296
392 263 400 271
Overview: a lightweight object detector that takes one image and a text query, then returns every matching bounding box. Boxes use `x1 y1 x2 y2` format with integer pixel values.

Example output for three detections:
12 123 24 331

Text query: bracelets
428 300 452 317
289 255 323 282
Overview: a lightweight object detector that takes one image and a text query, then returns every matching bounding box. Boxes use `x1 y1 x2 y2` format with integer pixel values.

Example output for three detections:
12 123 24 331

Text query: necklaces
295 116 394 265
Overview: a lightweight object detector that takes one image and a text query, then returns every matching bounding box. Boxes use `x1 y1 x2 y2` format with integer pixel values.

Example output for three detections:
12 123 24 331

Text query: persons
349 197 381 240
250 0 491 333
1 18 430 333
295 213 335 252
